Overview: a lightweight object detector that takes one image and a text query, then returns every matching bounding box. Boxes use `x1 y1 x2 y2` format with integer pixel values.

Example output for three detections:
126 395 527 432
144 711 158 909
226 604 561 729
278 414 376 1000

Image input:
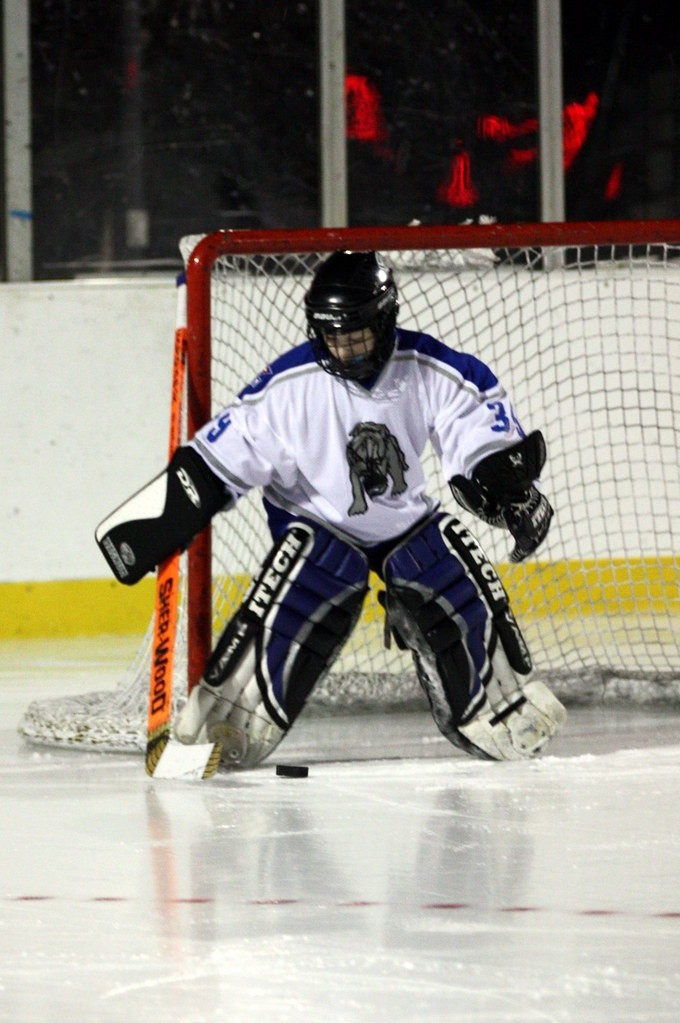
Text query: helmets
304 250 399 380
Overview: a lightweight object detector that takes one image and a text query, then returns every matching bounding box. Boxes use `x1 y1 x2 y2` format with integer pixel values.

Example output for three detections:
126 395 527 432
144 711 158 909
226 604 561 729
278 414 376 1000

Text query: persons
99 250 569 777
